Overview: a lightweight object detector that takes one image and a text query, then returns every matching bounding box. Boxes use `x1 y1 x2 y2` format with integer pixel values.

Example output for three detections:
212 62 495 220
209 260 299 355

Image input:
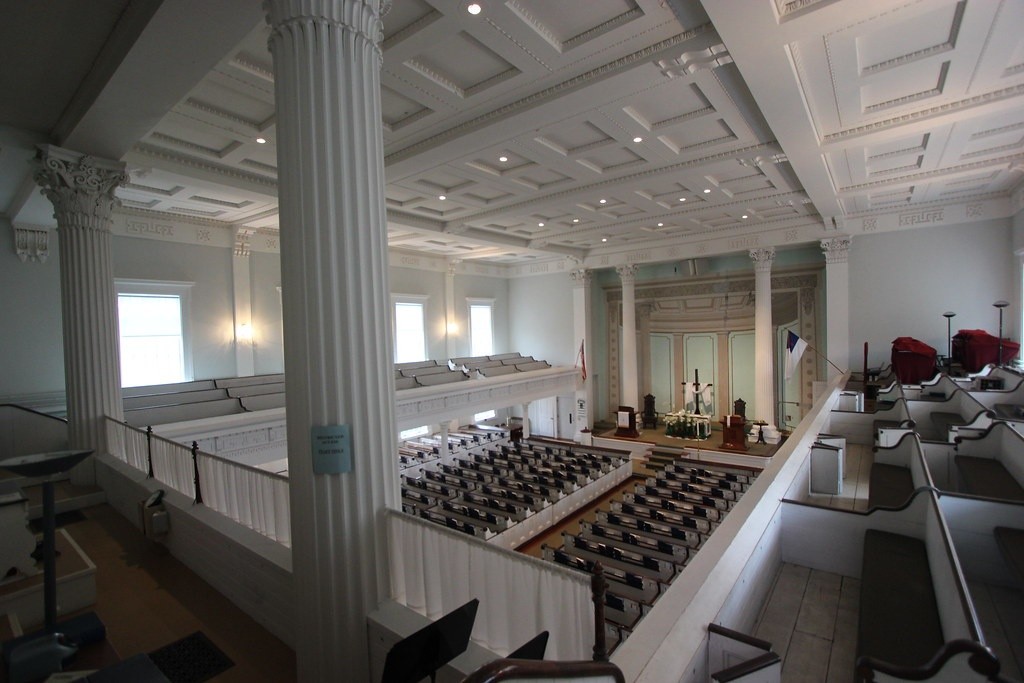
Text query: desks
752 422 769 445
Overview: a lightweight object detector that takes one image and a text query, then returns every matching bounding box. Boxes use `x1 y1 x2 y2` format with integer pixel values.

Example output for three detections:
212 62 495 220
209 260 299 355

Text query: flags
580 341 586 382
785 329 809 384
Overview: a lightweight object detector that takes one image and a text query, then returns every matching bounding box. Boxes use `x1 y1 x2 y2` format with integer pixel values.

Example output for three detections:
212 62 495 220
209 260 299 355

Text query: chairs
641 393 659 429
734 397 746 421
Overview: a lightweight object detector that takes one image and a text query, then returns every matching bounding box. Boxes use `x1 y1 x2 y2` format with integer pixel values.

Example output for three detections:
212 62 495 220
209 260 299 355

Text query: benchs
380 425 763 659
853 363 1024 683
122 353 553 429
